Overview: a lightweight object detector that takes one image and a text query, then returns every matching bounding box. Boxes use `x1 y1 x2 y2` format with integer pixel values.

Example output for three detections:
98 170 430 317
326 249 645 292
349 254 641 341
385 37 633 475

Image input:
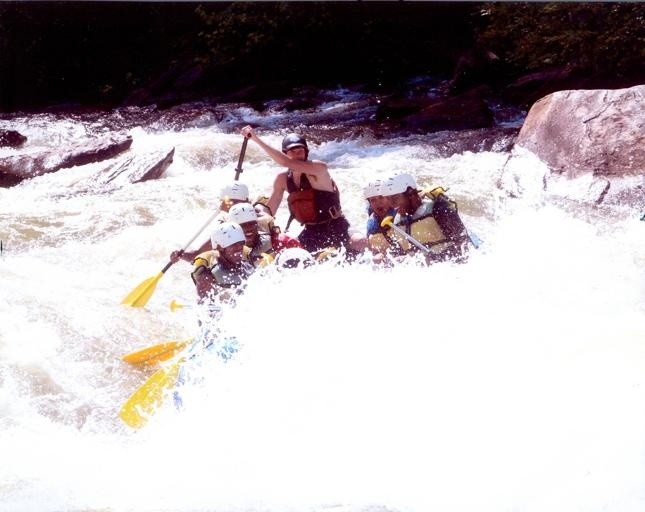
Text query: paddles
123 339 194 370
120 195 230 308
120 354 186 429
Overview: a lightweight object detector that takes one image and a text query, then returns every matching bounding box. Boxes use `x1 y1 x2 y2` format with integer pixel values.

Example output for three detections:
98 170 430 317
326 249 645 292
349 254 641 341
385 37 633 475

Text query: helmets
282 133 308 154
211 179 258 249
363 169 417 200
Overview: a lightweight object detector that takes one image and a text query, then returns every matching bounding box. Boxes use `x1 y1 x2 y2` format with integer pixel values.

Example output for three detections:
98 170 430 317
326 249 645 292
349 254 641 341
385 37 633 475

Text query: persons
241 126 365 253
364 171 473 263
172 183 301 298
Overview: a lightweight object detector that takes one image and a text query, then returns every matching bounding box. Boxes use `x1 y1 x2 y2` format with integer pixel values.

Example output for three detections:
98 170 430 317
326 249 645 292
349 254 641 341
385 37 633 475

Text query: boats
174 232 483 404
